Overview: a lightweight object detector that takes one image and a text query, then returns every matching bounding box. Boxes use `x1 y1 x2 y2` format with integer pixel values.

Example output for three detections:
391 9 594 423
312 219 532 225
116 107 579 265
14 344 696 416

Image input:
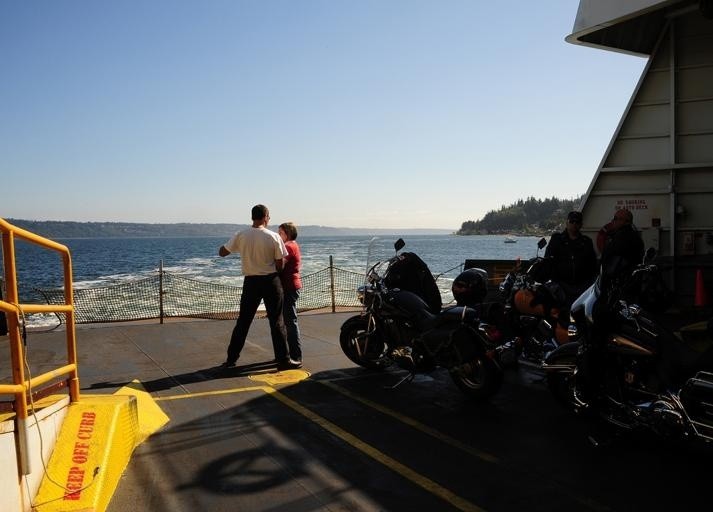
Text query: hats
568 212 583 221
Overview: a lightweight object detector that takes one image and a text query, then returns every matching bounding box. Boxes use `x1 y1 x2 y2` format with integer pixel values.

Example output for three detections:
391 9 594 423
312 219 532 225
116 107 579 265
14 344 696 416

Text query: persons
544 209 644 274
219 204 303 370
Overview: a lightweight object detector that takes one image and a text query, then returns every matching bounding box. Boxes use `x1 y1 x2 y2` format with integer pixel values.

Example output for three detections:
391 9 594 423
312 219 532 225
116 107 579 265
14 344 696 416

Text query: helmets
452 267 489 304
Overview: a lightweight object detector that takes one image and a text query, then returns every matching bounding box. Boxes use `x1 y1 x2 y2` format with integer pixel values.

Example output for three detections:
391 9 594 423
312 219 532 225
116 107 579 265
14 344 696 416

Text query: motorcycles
339 238 712 463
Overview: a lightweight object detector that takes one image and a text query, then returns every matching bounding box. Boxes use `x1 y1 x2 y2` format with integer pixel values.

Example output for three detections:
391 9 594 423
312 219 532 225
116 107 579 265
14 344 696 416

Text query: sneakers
222 357 303 371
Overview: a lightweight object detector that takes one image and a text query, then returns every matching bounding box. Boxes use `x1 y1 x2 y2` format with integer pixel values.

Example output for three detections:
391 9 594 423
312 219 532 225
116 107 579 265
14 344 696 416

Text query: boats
504 236 516 242
